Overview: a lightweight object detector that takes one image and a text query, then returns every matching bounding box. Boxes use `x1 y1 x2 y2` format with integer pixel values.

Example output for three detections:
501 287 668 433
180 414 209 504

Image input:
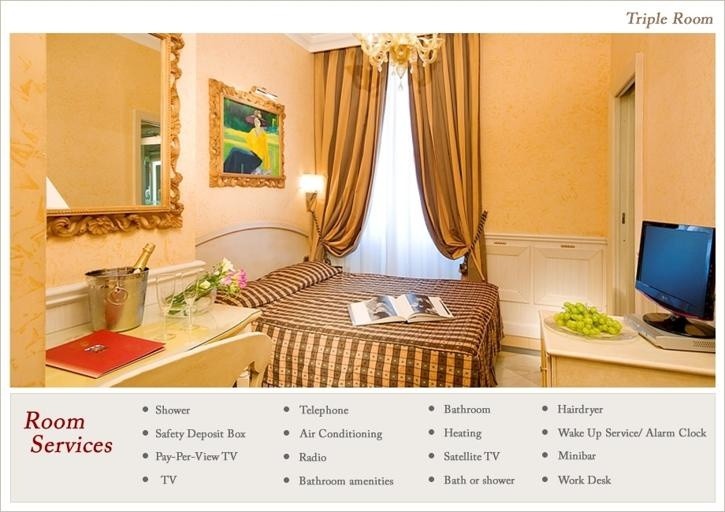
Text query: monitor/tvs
633 219 716 339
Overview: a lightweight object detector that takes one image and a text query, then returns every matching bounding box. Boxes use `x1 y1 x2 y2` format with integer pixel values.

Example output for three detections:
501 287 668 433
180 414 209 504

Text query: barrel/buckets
84 264 149 334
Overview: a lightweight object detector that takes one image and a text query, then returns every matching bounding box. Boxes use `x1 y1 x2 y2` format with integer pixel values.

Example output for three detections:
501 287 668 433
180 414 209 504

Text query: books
347 293 456 327
45 328 167 380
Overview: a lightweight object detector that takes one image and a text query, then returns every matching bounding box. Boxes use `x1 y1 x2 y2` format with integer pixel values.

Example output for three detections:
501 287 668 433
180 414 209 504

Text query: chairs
102 332 274 388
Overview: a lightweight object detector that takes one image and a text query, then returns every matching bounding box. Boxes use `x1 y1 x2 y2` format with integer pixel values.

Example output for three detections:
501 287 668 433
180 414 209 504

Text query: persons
223 114 271 175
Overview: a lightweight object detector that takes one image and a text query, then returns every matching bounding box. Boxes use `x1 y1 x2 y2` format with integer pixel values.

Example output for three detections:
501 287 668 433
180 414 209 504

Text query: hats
246 109 267 126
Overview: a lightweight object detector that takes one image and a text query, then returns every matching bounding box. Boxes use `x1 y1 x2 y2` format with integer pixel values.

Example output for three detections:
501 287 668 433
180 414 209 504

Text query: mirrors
47 33 184 238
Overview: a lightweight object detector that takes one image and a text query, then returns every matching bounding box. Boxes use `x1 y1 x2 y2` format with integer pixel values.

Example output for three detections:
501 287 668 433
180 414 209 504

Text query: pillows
215 262 342 308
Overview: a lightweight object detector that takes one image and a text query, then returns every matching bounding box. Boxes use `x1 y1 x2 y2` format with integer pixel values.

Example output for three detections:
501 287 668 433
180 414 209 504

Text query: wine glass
155 274 176 341
182 279 199 331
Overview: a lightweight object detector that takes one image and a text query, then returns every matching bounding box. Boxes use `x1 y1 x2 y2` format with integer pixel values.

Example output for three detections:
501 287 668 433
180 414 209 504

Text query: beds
195 220 504 387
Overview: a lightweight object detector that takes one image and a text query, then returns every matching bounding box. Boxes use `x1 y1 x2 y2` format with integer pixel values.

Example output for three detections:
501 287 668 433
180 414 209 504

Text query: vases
156 273 216 315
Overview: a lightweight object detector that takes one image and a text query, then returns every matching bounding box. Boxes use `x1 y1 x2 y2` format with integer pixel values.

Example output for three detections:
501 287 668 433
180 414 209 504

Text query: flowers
166 257 247 314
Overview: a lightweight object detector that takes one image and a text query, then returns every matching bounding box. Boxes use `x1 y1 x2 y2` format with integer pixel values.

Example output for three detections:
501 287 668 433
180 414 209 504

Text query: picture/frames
208 77 287 189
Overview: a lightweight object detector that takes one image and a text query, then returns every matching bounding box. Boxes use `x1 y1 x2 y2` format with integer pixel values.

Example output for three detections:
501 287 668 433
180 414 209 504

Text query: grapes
552 300 623 339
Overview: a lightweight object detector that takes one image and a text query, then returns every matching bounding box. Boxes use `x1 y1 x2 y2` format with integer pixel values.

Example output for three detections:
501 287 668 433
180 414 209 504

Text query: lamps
353 33 445 75
298 174 325 213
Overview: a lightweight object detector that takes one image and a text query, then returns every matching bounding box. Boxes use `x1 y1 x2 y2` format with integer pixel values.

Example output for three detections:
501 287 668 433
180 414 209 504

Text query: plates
543 314 637 344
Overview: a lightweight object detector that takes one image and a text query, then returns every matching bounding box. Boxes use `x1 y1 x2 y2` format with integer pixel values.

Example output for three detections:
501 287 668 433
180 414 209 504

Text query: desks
537 308 715 386
46 300 263 388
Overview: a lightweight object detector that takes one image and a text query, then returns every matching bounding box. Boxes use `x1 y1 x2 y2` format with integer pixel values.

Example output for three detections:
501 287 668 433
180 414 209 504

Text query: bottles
126 241 156 273
